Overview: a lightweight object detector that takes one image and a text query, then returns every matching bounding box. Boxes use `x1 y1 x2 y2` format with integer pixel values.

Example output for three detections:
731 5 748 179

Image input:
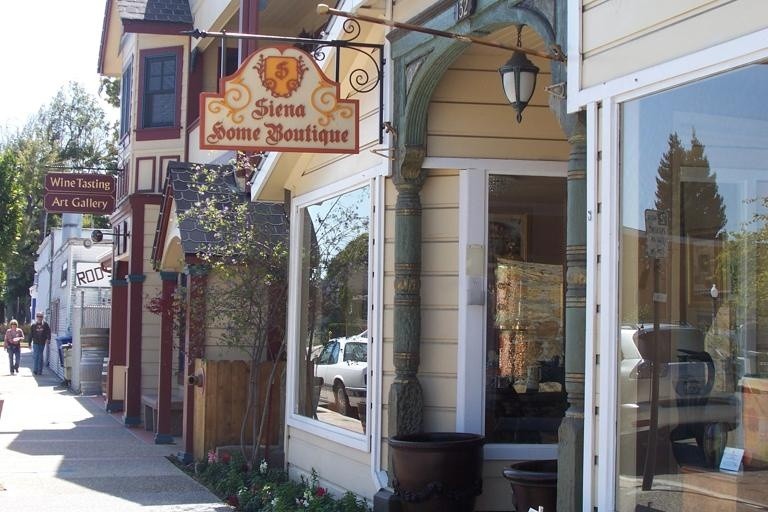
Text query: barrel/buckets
79 328 110 398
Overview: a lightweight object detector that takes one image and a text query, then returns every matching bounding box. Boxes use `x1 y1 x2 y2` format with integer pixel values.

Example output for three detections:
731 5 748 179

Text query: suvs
620 321 738 464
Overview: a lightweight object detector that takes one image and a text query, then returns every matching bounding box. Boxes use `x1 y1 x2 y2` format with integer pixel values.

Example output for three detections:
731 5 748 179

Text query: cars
308 336 368 415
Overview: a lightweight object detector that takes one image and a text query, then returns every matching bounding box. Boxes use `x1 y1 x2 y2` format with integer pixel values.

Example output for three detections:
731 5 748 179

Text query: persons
28 312 51 375
3 319 25 375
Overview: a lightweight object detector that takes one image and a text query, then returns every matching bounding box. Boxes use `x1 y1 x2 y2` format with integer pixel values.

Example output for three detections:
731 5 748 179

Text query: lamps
91 229 129 243
497 24 542 125
